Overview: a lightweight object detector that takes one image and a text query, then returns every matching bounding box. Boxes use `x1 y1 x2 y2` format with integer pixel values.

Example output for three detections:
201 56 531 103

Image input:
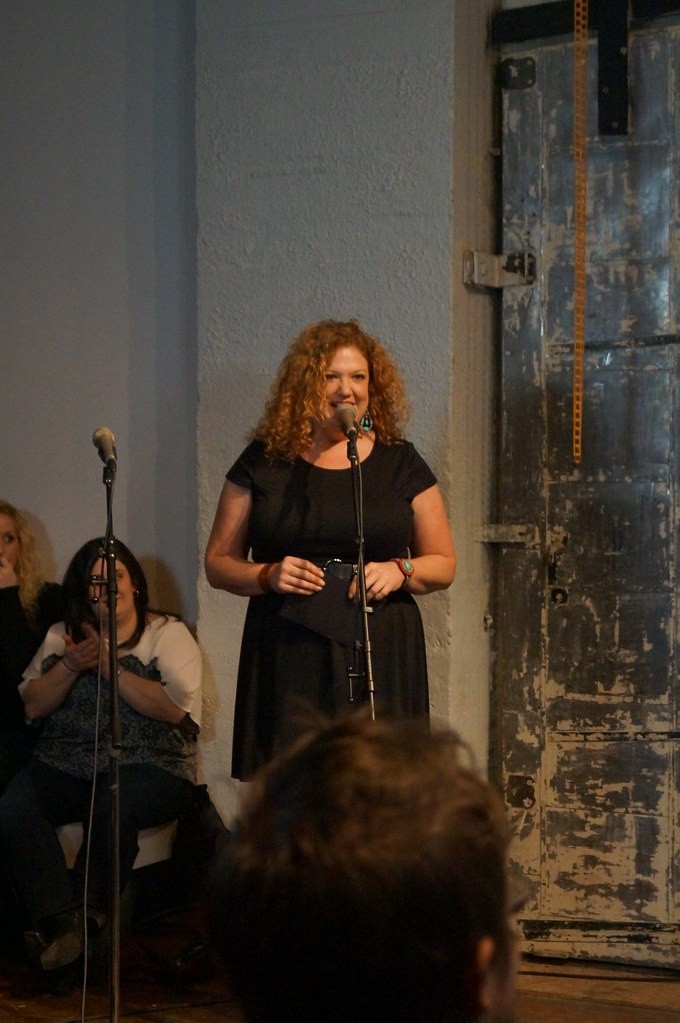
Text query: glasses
490 876 537 930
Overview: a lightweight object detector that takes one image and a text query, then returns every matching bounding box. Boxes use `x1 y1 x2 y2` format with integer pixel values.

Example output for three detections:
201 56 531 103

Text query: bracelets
103 662 129 683
258 562 274 595
63 659 83 672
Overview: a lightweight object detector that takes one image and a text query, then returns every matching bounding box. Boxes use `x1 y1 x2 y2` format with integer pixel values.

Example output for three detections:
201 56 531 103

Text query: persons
193 709 518 1023
0 500 61 795
203 319 458 782
0 536 234 997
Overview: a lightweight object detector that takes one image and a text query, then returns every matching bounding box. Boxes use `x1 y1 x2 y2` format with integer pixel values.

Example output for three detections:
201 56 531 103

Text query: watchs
389 558 415 591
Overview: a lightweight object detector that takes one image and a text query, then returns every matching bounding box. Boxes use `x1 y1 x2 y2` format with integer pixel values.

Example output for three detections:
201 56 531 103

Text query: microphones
336 403 358 438
93 428 118 472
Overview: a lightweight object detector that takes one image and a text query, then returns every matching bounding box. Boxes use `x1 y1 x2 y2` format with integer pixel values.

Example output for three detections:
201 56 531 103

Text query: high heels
40 904 106 971
26 929 83 996
